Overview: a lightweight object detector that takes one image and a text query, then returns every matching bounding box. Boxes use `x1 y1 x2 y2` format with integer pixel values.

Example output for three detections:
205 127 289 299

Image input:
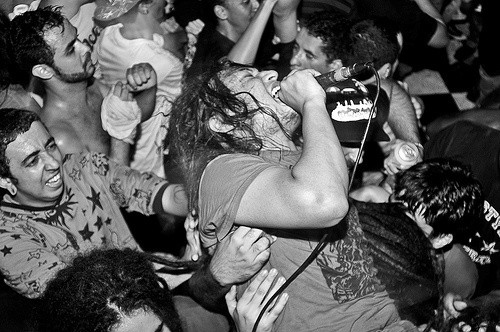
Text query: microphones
315 61 372 89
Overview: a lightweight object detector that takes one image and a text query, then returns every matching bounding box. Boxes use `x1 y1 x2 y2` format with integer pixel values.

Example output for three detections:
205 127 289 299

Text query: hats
94 0 140 21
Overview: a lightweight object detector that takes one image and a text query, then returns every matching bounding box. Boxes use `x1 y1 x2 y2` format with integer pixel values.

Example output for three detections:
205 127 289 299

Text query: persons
169 63 418 332
7 6 157 168
0 0 205 181
0 108 289 332
209 0 500 332
